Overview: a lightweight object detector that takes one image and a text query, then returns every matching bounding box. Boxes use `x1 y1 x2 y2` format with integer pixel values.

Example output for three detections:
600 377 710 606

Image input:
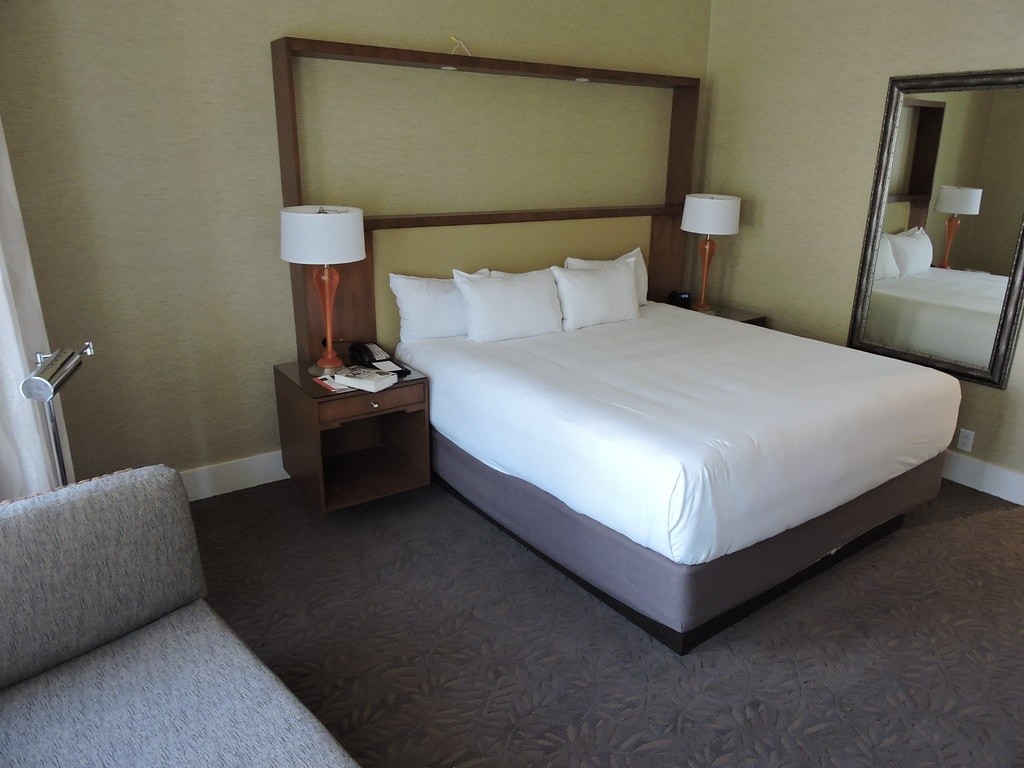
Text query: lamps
19 340 95 487
280 205 366 376
933 186 983 270
680 194 742 312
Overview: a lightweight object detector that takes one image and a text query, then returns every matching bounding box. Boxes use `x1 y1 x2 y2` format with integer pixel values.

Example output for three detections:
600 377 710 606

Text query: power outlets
957 429 975 453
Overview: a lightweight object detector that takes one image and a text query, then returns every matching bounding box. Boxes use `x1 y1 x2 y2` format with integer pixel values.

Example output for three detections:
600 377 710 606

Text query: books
335 366 398 393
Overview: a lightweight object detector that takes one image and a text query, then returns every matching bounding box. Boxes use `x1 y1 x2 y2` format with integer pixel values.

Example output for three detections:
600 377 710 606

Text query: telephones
349 341 391 368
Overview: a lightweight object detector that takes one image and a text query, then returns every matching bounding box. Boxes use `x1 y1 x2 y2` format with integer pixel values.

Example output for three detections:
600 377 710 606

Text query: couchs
0 463 364 768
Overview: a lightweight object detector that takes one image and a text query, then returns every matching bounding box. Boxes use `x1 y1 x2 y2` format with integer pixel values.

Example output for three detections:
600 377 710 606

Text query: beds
863 267 1009 368
394 298 961 655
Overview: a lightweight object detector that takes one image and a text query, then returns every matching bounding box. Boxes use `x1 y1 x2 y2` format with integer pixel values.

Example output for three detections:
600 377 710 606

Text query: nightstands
668 303 766 327
272 360 429 515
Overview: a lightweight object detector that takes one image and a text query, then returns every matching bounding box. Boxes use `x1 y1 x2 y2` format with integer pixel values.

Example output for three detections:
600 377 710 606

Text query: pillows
564 247 649 306
874 234 900 281
886 226 933 279
388 266 489 341
549 258 641 331
452 266 564 345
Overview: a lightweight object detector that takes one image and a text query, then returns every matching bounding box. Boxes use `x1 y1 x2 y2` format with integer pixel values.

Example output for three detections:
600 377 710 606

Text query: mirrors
846 68 1024 390
